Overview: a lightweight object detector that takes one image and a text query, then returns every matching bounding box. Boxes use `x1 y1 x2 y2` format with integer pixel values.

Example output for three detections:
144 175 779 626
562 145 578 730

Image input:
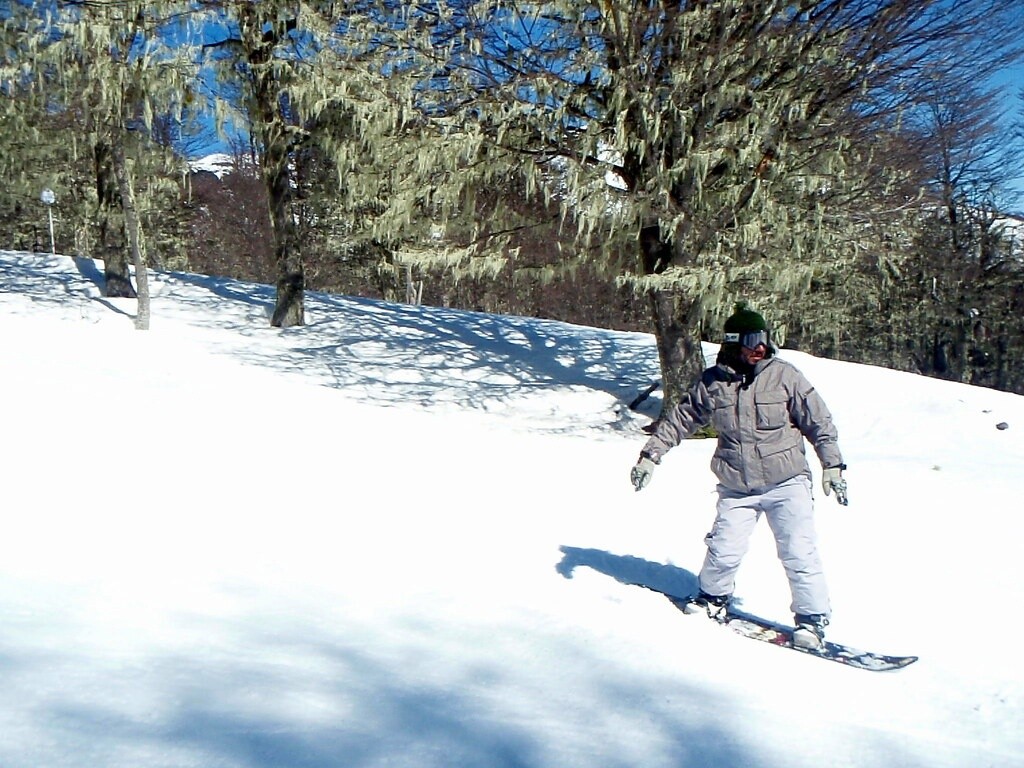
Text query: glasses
725 332 768 350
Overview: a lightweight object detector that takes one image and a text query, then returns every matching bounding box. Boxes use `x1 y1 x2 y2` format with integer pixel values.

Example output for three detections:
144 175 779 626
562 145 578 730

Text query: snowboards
714 601 919 672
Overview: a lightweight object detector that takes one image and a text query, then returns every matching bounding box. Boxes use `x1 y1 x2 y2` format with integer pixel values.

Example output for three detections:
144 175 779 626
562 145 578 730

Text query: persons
631 311 848 650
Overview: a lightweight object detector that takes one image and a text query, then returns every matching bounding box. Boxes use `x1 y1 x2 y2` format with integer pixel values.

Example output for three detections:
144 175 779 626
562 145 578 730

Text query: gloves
631 455 654 491
822 468 848 506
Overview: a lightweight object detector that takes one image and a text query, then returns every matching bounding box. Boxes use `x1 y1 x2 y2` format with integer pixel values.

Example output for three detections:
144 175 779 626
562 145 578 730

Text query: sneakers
793 612 827 650
685 593 728 619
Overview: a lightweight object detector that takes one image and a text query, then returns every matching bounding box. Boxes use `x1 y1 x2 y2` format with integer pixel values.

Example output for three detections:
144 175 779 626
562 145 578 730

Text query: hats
723 308 766 335
